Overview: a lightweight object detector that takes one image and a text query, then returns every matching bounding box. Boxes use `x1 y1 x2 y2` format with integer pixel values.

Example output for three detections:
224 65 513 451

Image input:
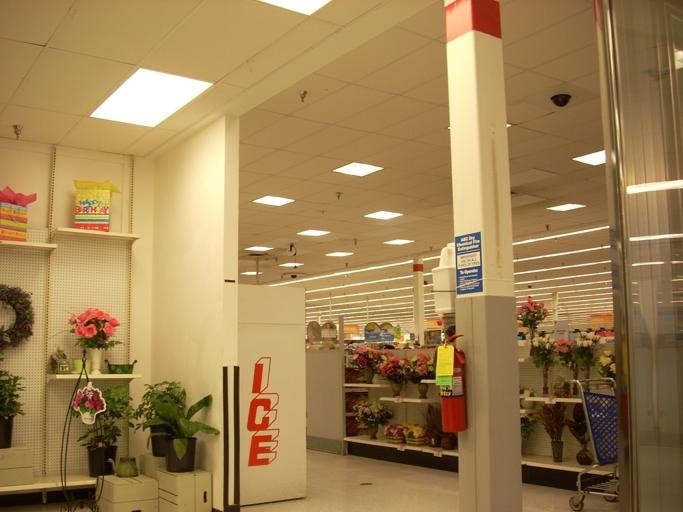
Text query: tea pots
108 457 139 477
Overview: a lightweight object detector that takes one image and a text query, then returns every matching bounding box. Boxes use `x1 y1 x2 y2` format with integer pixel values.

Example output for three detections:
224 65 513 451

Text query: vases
85 348 104 374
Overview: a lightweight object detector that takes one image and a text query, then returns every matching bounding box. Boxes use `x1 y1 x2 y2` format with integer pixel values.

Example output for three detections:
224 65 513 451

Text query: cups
74 359 90 373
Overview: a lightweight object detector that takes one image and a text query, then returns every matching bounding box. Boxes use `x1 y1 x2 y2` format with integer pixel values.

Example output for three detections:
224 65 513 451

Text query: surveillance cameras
550 94 571 107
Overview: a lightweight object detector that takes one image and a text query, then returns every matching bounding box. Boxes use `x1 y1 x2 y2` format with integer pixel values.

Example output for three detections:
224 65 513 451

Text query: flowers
516 297 549 340
0 284 35 354
67 308 123 350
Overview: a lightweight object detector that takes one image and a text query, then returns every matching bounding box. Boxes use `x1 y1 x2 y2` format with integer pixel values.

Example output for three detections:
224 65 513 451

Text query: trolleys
566 377 617 511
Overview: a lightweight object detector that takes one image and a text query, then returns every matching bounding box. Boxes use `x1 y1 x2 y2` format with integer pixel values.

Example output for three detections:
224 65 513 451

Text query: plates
307 322 394 343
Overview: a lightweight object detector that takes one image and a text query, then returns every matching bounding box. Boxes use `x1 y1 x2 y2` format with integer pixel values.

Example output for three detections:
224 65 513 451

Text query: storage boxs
0 201 29 242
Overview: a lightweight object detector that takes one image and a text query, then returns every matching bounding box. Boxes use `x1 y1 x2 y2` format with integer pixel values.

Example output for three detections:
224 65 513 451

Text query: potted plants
142 392 220 473
131 379 187 457
68 384 147 477
0 369 25 448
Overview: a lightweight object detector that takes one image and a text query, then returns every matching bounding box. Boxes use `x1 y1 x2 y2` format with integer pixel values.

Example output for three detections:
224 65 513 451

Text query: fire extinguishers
436 334 467 434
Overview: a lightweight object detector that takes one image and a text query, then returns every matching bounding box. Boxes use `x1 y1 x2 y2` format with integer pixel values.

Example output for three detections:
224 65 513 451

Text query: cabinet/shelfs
0 227 142 504
